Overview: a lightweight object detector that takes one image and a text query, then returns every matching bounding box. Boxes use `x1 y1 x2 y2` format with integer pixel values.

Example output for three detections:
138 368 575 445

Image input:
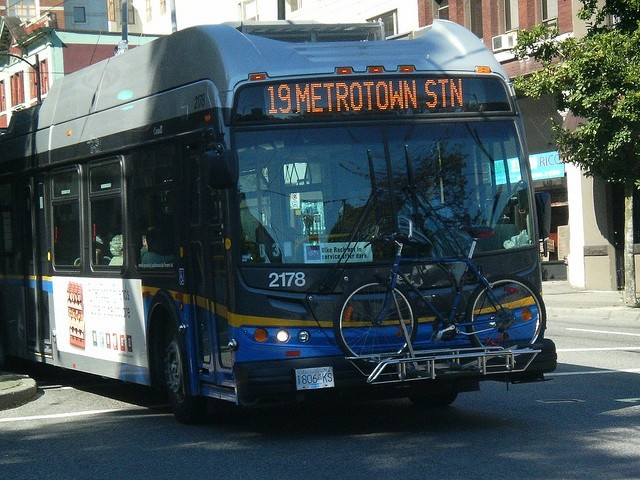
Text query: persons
143 201 176 230
395 158 462 241
108 234 123 266
96 223 107 264
177 193 199 257
140 230 177 264
140 229 148 257
548 225 557 248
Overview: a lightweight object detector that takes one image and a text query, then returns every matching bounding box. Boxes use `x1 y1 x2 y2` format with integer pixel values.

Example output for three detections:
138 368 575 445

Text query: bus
0 17 557 425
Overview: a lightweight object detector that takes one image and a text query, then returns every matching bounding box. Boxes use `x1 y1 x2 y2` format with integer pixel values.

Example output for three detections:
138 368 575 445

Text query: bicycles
332 224 546 370
